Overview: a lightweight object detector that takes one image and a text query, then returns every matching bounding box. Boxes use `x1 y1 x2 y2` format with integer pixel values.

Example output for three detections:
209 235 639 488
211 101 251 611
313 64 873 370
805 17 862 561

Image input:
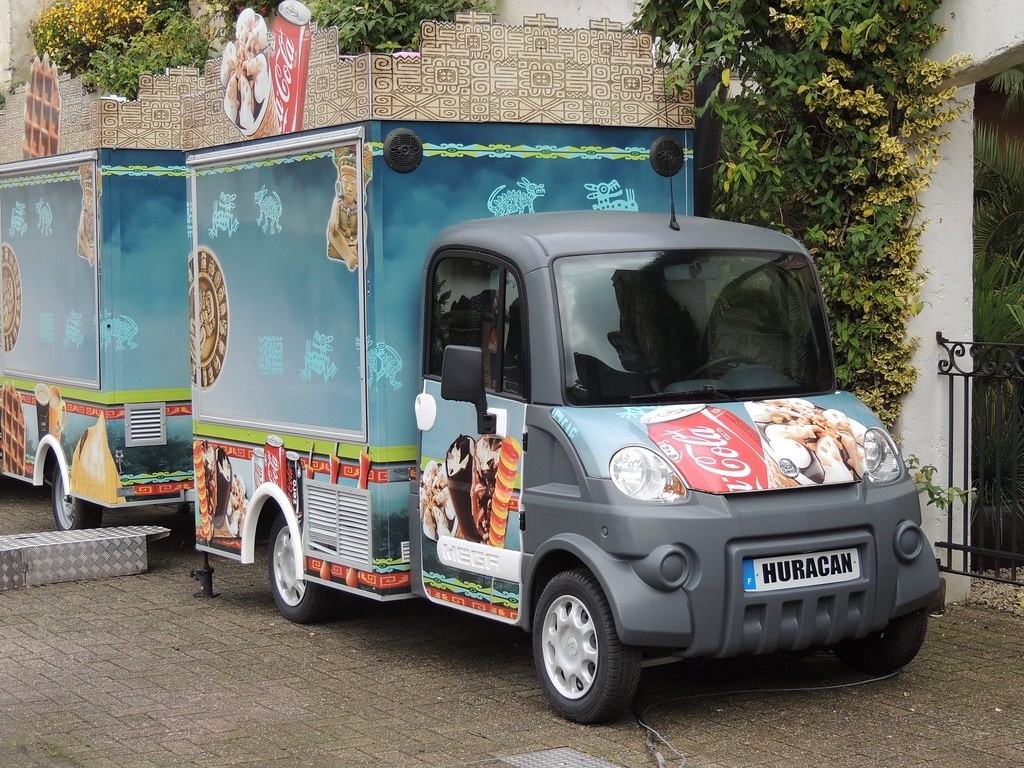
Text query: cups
49 386 67 443
35 383 51 442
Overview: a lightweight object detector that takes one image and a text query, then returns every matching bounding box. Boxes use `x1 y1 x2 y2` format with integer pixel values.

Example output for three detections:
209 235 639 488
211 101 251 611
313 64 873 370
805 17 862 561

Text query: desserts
745 399 868 487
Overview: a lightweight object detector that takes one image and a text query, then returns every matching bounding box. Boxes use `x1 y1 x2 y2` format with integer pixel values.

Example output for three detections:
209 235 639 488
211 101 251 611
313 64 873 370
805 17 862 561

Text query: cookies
1 382 26 476
22 52 61 159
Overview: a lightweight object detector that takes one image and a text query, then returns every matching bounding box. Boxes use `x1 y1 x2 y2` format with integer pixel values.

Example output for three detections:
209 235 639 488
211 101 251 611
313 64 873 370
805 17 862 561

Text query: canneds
264 435 286 492
270 1 311 134
640 404 769 492
286 451 302 515
252 449 264 493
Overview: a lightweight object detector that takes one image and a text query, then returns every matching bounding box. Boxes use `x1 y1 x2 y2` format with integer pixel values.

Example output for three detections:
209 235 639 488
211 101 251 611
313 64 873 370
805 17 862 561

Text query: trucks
185 117 942 726
0 145 194 533
